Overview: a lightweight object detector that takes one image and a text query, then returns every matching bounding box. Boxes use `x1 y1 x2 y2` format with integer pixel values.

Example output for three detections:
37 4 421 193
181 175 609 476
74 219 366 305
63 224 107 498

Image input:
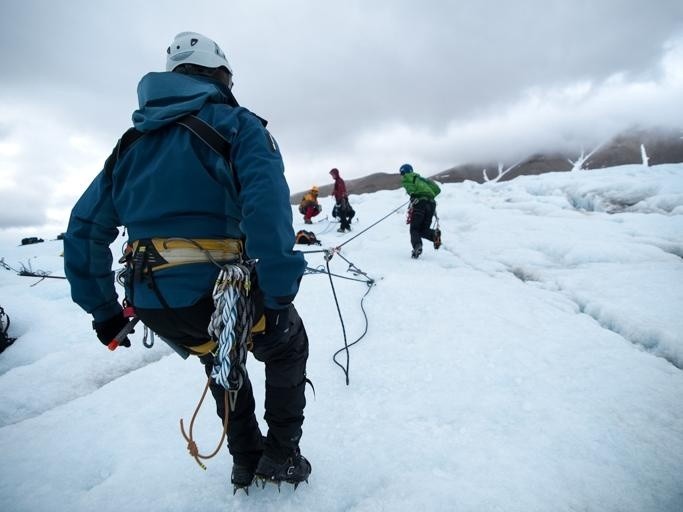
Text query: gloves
92 312 134 347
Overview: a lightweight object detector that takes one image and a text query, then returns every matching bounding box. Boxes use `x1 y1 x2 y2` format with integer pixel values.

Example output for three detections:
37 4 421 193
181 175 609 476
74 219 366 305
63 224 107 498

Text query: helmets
312 187 318 192
165 32 234 77
400 165 412 174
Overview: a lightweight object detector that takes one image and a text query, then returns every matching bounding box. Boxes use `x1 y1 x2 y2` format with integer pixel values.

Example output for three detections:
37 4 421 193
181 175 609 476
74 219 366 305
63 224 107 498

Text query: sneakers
337 227 351 233
304 217 312 224
412 245 422 259
434 230 441 249
230 436 312 496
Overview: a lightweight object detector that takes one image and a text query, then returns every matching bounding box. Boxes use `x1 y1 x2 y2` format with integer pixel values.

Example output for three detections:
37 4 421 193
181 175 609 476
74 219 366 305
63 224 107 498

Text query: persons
329 168 355 232
399 163 442 259
65 34 312 487
299 187 322 224
